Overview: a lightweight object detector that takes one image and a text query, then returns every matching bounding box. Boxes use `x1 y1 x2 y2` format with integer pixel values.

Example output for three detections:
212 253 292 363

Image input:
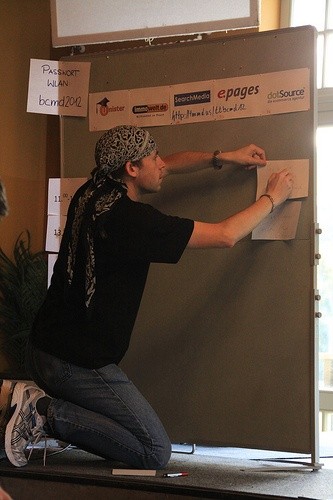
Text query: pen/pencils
163 473 190 478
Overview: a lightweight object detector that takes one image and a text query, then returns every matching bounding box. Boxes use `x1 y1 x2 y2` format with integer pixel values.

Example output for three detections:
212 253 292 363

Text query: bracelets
212 149 224 170
258 194 275 215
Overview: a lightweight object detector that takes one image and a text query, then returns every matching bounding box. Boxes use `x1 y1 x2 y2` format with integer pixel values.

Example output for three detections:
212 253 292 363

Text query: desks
0 445 333 500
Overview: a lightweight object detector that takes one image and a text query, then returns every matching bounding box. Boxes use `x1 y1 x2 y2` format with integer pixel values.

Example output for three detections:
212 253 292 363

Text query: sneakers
0 380 17 437
5 381 45 467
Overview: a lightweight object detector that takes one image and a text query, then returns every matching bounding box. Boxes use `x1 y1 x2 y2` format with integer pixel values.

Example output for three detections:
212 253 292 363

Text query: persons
0 124 295 472
